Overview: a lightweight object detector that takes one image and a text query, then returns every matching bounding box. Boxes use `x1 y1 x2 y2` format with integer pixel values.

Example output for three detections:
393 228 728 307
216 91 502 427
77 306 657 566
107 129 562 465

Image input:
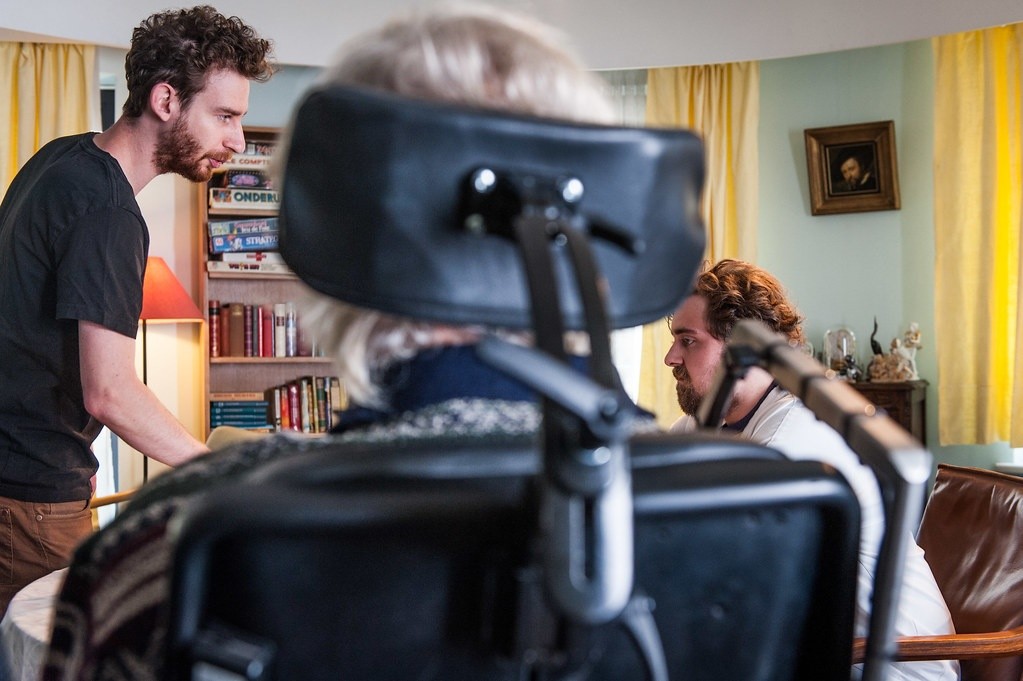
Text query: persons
663 261 963 681
830 147 880 193
0 4 281 619
34 10 680 681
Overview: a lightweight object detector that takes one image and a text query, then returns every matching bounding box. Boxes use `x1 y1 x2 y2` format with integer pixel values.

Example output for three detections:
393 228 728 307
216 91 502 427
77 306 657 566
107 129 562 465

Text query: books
209 141 347 439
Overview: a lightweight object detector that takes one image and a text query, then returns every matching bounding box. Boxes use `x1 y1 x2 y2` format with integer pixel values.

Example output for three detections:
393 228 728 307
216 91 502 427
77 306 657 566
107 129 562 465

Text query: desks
0 567 68 681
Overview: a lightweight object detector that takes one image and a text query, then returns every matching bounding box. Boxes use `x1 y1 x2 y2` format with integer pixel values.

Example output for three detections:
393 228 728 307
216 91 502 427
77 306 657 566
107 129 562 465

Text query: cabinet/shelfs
193 125 351 444
855 379 929 449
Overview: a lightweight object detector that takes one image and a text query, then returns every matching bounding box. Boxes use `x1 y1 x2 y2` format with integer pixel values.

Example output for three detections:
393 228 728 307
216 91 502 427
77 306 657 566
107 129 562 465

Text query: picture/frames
804 120 901 216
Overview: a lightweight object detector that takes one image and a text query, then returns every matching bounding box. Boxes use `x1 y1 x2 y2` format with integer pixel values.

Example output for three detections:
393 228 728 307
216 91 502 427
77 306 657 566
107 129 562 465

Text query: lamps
139 256 205 485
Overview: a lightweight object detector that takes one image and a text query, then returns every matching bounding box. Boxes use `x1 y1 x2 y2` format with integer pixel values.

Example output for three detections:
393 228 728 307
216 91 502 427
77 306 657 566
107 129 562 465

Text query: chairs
852 463 1023 681
38 84 864 681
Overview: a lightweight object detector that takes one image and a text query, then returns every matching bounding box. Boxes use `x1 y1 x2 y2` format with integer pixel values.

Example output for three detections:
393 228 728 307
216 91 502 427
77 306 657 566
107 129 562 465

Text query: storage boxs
206 142 289 274
